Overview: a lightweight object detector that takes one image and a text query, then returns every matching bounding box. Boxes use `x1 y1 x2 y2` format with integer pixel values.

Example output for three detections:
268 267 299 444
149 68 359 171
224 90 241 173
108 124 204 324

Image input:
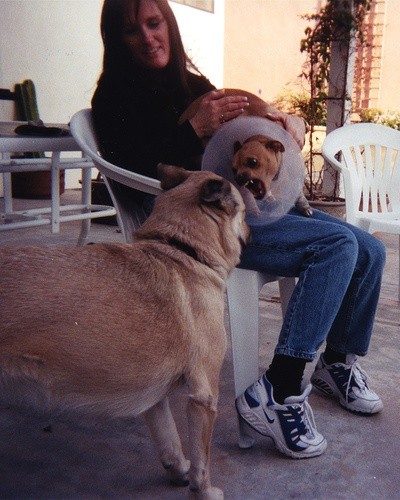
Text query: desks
0 121 116 245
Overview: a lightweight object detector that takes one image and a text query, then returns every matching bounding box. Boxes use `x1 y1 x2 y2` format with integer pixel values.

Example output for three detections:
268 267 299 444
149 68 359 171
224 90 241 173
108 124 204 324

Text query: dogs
177 86 318 219
0 159 252 500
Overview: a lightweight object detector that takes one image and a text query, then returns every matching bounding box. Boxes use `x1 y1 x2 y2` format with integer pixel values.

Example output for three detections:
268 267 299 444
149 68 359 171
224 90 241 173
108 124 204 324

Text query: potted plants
288 0 372 221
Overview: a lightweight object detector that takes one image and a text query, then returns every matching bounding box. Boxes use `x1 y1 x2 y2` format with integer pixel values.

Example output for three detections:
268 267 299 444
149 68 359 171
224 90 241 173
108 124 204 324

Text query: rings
220 114 225 122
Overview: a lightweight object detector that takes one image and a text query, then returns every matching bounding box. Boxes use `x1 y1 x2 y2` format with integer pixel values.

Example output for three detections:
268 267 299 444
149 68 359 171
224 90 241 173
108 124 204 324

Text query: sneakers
310 353 384 415
235 372 328 459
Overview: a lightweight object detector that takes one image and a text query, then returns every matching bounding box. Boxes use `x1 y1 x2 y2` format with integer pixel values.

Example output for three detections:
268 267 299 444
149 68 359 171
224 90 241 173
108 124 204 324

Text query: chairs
69 108 298 448
320 122 400 236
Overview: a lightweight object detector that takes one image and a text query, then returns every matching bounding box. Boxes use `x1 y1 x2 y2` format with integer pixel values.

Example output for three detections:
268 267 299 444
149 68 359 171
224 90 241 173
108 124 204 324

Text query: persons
90 0 386 460
